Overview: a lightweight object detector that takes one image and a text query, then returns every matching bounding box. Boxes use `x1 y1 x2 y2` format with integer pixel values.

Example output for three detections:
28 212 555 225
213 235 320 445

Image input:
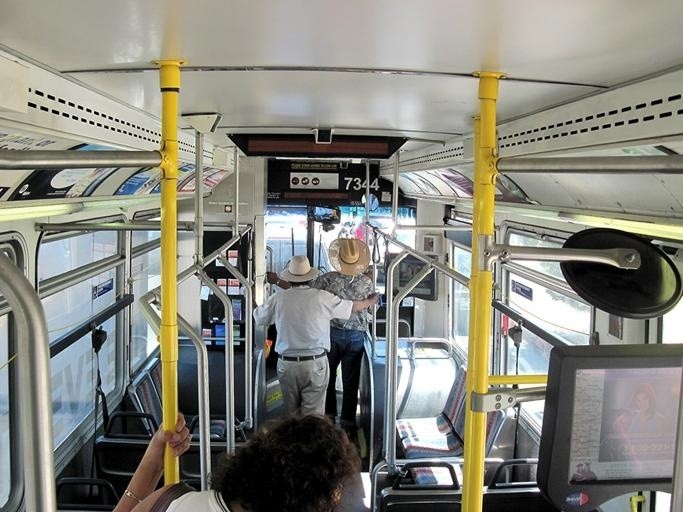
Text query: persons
267 238 374 440
612 382 667 434
242 254 380 419
110 411 363 512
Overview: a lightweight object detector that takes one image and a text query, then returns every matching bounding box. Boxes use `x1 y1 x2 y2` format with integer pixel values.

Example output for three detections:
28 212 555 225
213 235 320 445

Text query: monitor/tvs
385 253 438 301
536 342 683 512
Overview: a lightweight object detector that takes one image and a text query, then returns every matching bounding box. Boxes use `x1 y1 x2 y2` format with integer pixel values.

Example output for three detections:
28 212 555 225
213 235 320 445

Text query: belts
278 352 325 361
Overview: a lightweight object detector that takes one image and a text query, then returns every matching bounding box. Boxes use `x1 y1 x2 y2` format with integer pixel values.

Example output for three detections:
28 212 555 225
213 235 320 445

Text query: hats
280 255 317 282
328 238 371 275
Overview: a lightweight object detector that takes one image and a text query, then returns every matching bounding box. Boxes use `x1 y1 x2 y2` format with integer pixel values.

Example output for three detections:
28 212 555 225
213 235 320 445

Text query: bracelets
123 488 142 504
276 279 280 286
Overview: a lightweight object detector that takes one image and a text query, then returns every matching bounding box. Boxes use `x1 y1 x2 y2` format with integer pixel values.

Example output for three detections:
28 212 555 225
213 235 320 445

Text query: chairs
395 365 507 487
55 357 245 511
